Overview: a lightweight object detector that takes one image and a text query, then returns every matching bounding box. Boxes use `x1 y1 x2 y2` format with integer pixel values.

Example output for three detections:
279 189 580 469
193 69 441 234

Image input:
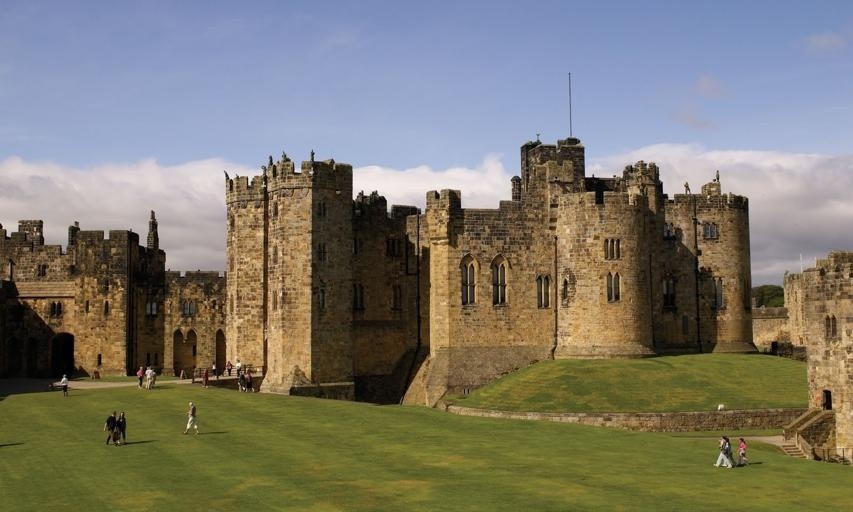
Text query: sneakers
106 442 126 446
184 432 199 434
714 463 735 468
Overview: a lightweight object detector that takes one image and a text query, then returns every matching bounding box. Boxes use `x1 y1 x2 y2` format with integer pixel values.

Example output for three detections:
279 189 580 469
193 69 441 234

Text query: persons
713 169 721 182
101 409 118 448
684 180 692 194
182 400 201 436
711 436 733 469
43 355 259 397
736 438 748 467
722 438 736 467
116 410 127 447
